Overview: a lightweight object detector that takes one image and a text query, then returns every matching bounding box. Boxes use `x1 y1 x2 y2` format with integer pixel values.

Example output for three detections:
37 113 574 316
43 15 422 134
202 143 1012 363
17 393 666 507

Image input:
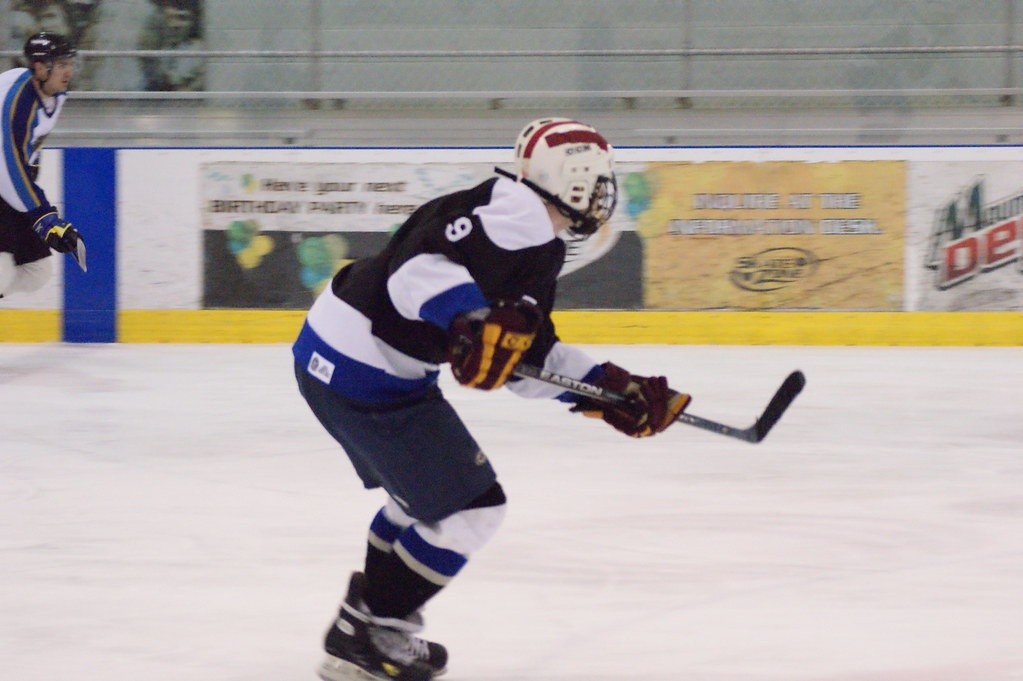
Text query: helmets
514 118 618 241
24 33 83 81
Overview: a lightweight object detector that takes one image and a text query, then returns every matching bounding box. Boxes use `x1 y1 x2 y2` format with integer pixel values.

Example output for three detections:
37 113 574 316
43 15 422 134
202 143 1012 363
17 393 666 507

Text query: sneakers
319 572 448 681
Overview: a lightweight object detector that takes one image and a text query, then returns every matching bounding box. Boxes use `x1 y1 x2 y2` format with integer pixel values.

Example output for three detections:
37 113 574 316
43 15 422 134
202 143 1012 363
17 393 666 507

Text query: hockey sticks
51 204 88 274
450 343 807 446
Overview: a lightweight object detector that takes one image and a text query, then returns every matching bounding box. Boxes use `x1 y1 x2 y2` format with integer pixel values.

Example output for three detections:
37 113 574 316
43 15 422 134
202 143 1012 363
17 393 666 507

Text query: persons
12 0 206 92
290 118 671 681
0 30 88 299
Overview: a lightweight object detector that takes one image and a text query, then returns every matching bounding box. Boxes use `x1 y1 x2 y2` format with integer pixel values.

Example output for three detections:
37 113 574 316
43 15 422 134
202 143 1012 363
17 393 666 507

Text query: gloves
569 362 692 439
449 300 542 392
30 206 83 253
